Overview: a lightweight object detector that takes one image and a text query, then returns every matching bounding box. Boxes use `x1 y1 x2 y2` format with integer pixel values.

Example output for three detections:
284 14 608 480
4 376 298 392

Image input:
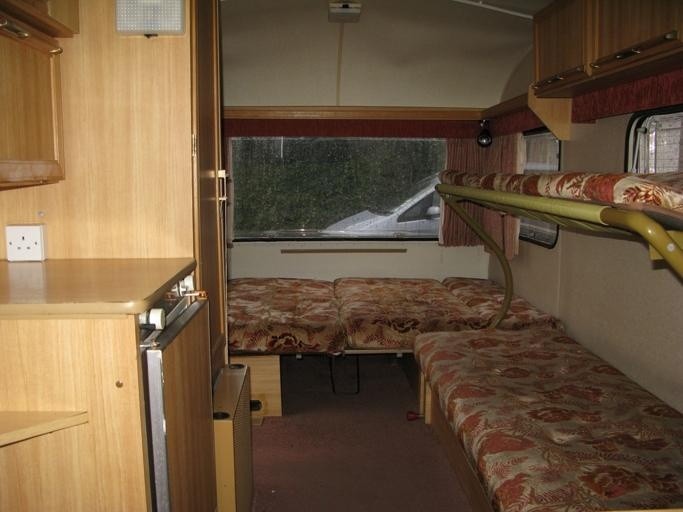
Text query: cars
320 163 557 238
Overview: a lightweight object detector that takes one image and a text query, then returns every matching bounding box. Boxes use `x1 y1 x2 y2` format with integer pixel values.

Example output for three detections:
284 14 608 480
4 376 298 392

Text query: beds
227 273 561 357
407 328 682 512
440 167 683 324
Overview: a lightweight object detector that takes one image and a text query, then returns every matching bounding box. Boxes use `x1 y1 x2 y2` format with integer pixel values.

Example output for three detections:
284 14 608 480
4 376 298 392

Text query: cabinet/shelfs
531 0 681 99
0 11 63 184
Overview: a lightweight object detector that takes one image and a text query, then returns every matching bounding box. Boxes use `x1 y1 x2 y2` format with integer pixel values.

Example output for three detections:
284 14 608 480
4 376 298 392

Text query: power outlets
4 222 47 263
